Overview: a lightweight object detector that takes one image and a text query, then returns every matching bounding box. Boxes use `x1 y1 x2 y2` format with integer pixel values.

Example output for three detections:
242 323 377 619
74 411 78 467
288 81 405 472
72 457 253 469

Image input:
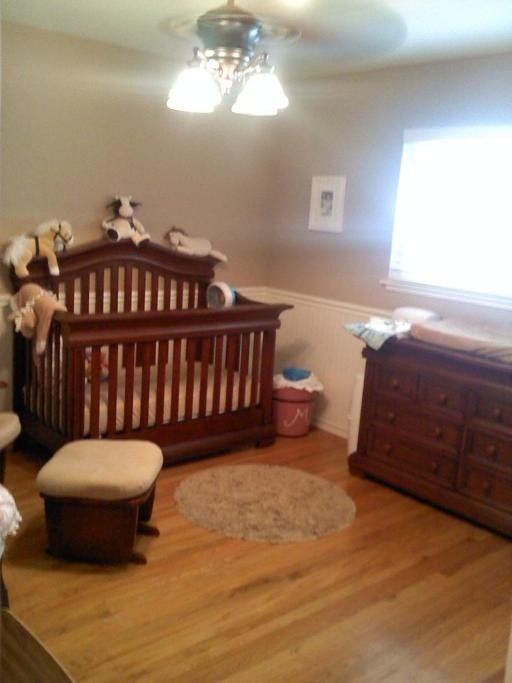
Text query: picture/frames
307 175 346 232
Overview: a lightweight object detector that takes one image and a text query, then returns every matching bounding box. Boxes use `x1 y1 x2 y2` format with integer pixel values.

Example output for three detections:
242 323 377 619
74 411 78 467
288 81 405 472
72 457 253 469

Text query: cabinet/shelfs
346 331 511 543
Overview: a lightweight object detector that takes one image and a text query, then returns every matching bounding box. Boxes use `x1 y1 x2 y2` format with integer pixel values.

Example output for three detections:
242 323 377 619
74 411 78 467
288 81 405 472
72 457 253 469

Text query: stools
35 438 164 566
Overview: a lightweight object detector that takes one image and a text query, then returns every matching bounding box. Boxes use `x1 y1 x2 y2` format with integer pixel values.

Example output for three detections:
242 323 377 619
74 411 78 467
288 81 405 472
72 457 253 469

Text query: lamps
164 1 289 120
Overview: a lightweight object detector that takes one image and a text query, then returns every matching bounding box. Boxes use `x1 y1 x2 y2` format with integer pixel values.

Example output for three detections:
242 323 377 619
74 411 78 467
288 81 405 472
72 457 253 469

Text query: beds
9 238 296 473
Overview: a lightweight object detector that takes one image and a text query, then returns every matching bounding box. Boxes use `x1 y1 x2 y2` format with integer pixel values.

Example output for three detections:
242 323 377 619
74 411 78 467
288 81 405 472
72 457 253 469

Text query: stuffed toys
164 226 229 264
7 281 68 354
3 219 74 279
101 194 151 250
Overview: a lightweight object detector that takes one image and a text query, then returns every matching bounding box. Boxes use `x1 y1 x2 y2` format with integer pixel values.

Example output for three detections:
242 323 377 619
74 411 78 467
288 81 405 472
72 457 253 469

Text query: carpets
172 464 356 543
